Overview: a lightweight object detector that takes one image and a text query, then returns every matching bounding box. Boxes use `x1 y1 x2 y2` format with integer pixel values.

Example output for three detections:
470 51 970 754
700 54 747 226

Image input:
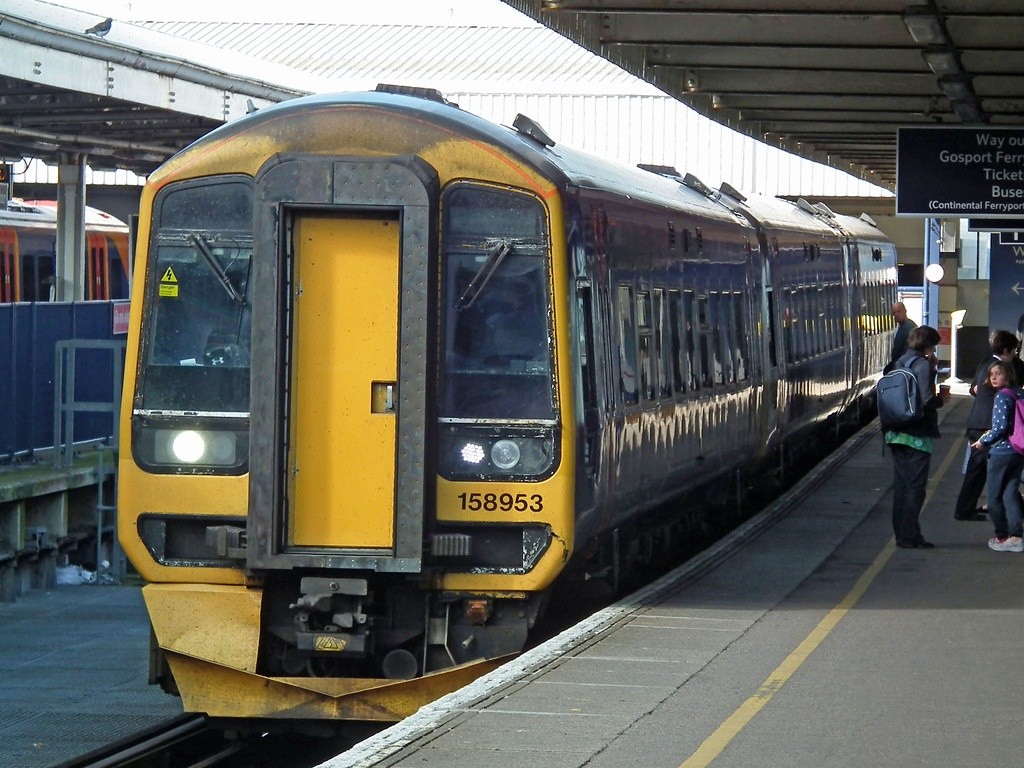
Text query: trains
113 84 902 738
0 194 131 303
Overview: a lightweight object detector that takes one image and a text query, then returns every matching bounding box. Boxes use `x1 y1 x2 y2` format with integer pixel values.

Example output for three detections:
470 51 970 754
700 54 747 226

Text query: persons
954 314 1024 552
883 301 951 549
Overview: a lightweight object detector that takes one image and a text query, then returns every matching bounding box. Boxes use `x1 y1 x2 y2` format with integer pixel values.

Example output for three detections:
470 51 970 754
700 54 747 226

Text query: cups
939 384 950 394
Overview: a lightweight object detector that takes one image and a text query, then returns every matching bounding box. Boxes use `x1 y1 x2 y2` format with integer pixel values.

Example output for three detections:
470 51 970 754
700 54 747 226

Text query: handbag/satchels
1015 341 1022 359
962 440 972 474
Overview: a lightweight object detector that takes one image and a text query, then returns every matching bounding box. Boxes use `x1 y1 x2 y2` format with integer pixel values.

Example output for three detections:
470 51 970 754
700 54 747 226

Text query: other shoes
955 510 986 521
896 541 934 549
978 504 990 513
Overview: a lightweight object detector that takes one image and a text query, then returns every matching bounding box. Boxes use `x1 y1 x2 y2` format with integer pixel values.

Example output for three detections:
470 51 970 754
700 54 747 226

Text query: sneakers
992 537 1023 552
988 536 1010 548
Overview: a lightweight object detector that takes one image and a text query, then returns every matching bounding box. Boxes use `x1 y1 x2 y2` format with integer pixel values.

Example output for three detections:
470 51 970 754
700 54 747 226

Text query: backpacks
999 388 1024 455
877 356 924 433
